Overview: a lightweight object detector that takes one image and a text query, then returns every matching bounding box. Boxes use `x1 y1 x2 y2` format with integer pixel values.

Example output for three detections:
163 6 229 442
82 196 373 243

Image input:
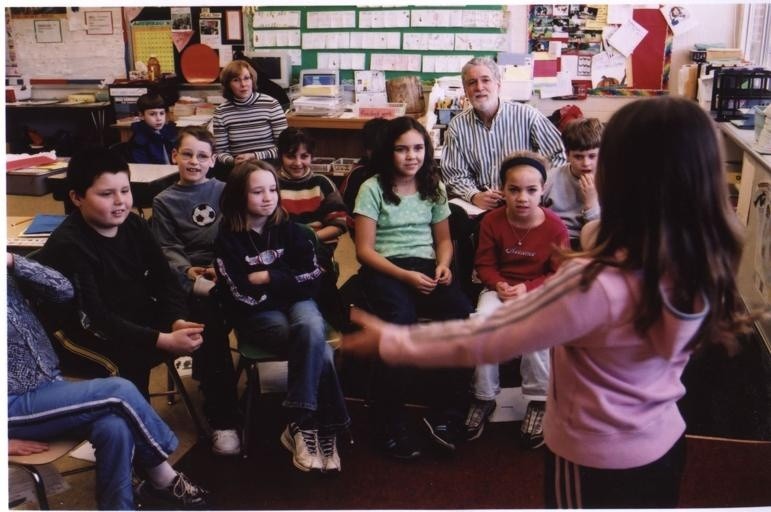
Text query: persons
6 59 602 508
330 97 747 509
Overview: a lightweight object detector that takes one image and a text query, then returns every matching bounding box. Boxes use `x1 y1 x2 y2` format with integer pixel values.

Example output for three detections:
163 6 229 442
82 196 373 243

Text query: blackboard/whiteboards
5 7 742 98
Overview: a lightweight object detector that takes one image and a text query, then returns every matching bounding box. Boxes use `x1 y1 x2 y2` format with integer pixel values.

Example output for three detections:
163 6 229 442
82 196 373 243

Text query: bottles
147 54 160 82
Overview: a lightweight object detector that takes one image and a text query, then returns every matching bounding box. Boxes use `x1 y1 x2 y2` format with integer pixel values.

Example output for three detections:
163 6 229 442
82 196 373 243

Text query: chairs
25 248 203 434
235 244 355 457
9 439 84 510
449 204 474 294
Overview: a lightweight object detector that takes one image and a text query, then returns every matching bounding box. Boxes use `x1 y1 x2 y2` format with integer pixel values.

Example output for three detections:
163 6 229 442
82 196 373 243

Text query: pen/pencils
435 97 458 109
483 184 493 193
551 96 580 100
12 218 32 227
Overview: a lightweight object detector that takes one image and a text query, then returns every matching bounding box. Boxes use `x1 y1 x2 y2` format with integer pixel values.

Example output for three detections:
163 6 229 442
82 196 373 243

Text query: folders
23 215 69 235
729 119 755 129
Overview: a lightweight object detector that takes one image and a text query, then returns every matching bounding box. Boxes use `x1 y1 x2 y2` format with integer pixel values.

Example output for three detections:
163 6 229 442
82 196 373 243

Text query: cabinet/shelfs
720 121 771 355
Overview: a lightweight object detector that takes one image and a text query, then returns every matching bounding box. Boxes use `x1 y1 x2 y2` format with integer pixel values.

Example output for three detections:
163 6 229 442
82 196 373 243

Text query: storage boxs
6 156 71 196
48 162 179 208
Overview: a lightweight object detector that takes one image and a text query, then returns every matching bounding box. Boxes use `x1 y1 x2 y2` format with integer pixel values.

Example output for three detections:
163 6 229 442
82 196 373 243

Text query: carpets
174 393 769 507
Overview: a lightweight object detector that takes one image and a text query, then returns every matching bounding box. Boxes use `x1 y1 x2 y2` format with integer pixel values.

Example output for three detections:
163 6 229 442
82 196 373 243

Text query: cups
573 85 588 100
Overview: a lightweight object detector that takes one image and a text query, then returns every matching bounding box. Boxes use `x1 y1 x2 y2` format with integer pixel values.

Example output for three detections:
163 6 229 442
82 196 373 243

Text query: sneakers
320 435 341 472
377 434 421 458
519 400 545 450
461 400 496 441
212 430 240 456
422 414 456 452
281 422 323 473
148 472 215 507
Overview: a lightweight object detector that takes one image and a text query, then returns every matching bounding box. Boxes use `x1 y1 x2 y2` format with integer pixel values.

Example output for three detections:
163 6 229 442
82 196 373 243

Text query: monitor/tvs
247 51 292 89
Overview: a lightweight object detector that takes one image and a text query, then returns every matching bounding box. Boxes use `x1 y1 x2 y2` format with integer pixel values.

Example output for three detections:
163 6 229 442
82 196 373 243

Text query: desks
6 99 111 130
111 115 214 142
7 194 153 255
525 95 639 123
109 78 222 120
285 109 365 160
